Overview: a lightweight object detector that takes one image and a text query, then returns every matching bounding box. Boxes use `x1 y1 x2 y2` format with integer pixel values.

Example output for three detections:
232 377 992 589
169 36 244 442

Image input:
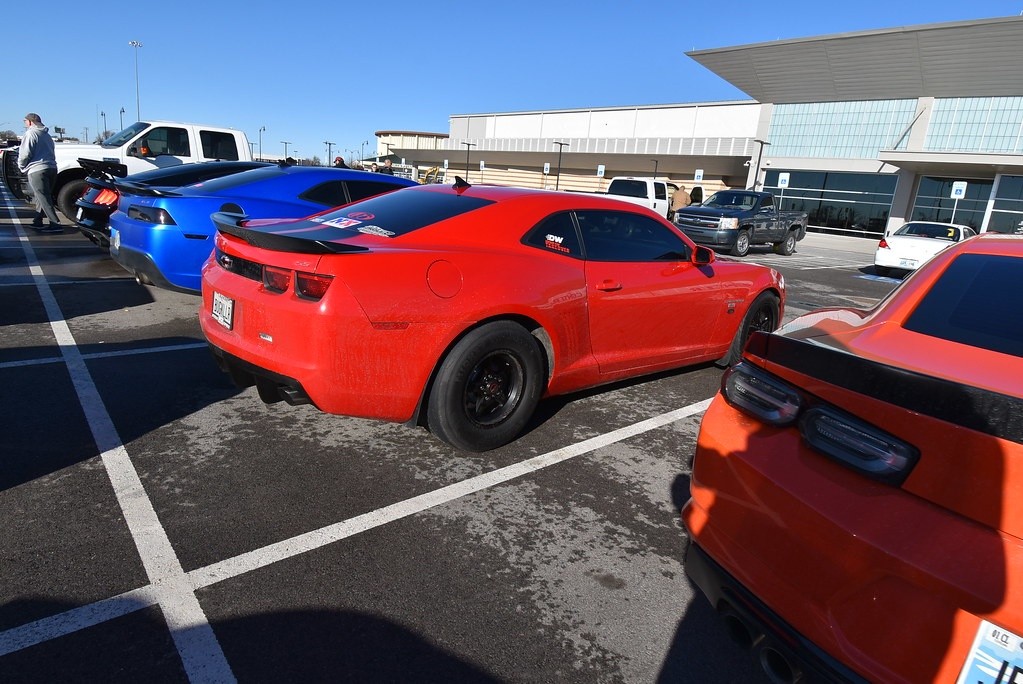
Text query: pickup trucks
563 176 703 222
671 189 808 257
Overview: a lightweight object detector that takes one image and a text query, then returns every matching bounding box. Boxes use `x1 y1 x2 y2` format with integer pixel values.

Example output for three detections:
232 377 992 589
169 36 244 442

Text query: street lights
118 107 125 130
323 142 336 166
259 126 266 161
326 149 339 163
101 110 108 139
345 149 359 166
749 140 771 205
461 142 476 184
362 141 369 167
553 142 569 191
129 40 143 122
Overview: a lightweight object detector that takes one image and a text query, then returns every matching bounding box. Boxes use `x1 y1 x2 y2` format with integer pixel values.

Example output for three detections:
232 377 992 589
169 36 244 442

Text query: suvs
52 120 254 223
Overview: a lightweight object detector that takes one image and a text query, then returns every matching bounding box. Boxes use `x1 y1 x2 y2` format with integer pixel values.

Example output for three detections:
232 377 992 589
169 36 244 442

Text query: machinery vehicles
417 166 439 185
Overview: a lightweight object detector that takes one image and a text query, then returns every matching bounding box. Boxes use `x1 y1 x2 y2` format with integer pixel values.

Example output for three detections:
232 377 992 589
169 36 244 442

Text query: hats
333 157 342 163
24 113 43 126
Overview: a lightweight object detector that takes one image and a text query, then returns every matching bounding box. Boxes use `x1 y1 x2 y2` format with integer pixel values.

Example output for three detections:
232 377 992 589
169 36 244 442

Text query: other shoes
27 220 43 229
41 222 63 232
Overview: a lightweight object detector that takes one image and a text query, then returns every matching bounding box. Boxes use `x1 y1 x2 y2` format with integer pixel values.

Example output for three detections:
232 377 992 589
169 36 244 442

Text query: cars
74 158 277 253
0 146 20 159
0 141 14 148
200 177 785 453
683 232 1023 684
874 221 978 276
107 160 441 297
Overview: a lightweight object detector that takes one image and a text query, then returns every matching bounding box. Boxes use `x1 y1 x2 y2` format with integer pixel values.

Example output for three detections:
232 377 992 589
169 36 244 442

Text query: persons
17 113 65 232
334 157 351 168
671 185 691 223
371 159 394 175
